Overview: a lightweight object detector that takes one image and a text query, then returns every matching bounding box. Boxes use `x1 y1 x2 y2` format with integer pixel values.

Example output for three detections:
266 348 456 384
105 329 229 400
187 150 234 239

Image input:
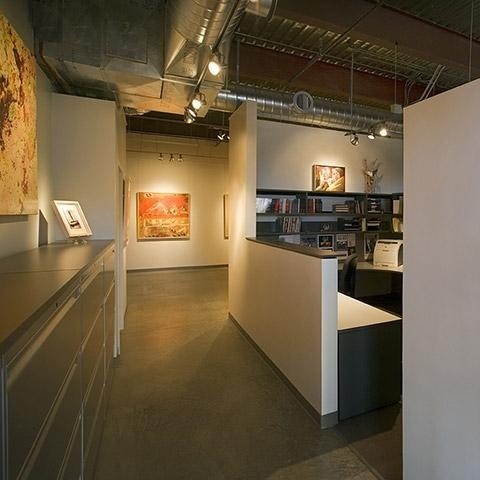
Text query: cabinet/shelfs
0 236 118 479
255 187 403 263
336 290 402 421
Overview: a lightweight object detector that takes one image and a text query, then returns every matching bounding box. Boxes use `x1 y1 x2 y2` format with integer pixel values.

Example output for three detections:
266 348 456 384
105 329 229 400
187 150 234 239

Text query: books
271 197 383 232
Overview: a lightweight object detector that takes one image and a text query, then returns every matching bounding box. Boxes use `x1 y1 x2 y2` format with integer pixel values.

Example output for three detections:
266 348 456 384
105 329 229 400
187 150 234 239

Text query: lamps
343 41 399 147
215 74 233 142
182 1 242 126
362 165 380 194
157 152 184 163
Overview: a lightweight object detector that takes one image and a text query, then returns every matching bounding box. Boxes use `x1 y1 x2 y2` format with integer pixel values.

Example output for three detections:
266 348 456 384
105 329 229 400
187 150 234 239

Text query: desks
337 259 403 301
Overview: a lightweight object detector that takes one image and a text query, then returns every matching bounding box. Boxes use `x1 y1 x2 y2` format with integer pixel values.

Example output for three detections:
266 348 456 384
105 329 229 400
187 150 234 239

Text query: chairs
337 253 360 299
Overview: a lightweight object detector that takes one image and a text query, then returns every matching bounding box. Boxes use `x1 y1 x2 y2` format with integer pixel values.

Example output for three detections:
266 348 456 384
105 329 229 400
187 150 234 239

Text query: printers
373 239 403 267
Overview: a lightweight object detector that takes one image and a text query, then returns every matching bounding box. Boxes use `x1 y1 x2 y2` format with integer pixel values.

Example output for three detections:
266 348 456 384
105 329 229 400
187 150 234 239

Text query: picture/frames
223 194 229 240
135 191 192 241
316 234 350 251
50 199 93 239
311 164 346 193
